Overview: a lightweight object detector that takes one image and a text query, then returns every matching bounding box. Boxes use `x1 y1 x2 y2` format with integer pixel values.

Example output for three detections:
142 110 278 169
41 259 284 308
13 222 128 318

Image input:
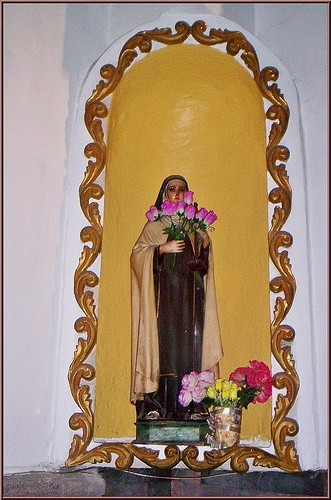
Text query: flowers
179 358 274 411
145 190 218 270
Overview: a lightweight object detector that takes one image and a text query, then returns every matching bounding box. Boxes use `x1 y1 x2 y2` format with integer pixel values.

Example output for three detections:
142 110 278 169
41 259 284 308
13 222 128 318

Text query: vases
212 407 246 449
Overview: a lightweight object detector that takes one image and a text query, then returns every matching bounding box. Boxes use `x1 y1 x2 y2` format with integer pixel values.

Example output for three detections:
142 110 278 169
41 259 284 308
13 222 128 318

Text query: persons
128 173 225 425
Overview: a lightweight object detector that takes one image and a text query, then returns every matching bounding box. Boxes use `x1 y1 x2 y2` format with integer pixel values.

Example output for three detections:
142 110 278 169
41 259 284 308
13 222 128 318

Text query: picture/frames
0 0 331 500
